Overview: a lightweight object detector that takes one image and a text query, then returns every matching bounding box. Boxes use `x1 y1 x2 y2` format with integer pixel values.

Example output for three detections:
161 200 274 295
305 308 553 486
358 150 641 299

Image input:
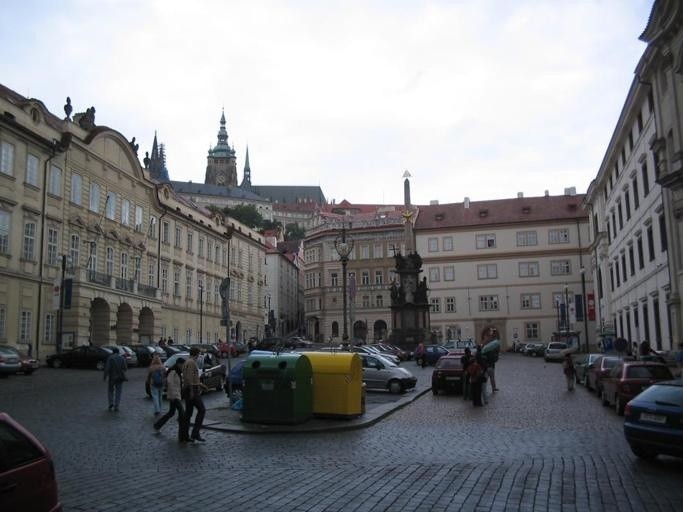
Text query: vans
441 340 476 353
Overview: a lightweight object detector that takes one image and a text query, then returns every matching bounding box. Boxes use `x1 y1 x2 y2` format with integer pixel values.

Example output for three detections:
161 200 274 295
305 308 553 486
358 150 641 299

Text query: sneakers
155 411 161 415
492 389 499 393
109 404 119 412
153 425 205 443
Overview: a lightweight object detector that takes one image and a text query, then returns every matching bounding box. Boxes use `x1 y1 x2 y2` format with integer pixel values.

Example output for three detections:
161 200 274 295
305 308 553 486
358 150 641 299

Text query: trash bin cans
238 349 362 425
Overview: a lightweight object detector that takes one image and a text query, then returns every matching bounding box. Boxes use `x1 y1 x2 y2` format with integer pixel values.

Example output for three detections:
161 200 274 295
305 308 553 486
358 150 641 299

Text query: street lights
579 266 589 353
334 218 353 346
556 281 569 335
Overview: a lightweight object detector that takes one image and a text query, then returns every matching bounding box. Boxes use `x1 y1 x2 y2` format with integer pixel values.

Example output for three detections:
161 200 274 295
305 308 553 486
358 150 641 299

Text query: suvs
223 360 244 405
0 411 61 512
520 335 682 459
0 346 39 376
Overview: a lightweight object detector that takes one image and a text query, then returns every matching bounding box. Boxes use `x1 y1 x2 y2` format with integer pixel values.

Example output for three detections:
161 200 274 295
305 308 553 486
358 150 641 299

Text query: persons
181 345 206 443
639 341 660 359
460 339 502 407
103 348 128 412
415 339 427 368
152 357 188 442
148 352 165 415
562 349 576 391
630 341 639 360
676 342 682 380
158 334 175 346
387 249 429 307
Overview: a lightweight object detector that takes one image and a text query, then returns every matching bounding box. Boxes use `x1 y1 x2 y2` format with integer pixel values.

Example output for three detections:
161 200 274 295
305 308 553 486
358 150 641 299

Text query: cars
45 336 312 370
320 345 470 394
145 353 226 399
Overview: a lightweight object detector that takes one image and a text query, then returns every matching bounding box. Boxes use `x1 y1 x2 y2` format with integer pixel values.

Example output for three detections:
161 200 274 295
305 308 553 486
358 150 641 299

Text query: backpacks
152 369 163 388
